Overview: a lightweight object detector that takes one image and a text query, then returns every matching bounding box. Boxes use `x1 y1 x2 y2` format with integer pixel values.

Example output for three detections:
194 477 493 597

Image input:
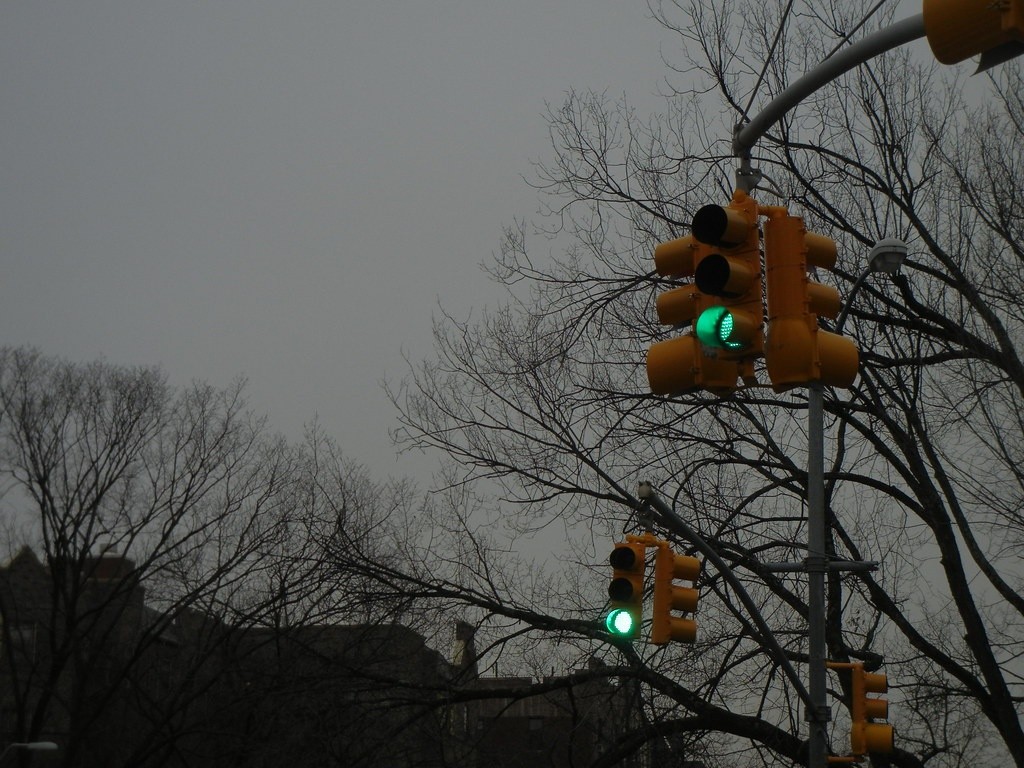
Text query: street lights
801 235 908 767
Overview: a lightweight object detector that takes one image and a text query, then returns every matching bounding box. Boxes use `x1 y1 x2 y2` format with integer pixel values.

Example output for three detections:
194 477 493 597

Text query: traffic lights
850 663 894 756
759 214 860 395
652 547 702 646
646 231 740 395
606 544 646 641
690 196 765 364
